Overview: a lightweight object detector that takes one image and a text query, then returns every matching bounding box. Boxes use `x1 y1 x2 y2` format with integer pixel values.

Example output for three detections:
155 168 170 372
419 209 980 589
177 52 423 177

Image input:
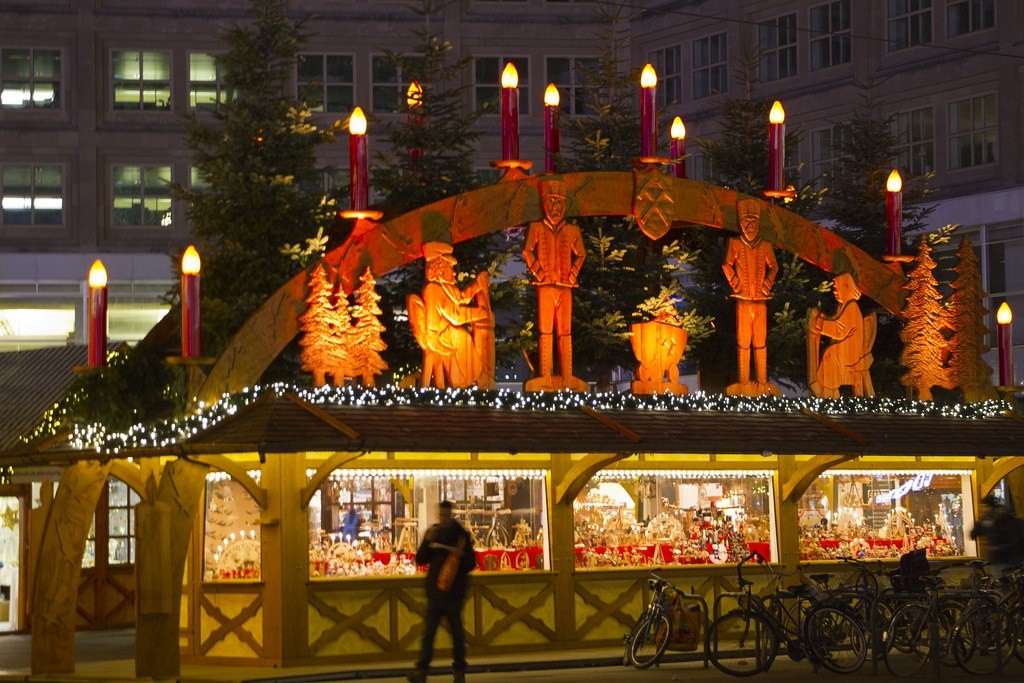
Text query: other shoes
453 672 466 683
406 669 428 683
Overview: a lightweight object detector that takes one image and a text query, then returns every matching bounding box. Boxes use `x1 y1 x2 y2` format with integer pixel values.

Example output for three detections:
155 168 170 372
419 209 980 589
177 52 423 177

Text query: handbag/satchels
437 536 466 591
899 547 932 593
656 595 701 652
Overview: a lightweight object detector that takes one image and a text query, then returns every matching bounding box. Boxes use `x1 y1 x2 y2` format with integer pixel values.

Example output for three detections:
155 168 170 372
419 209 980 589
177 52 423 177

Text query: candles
499 62 521 160
767 101 786 190
671 115 686 178
87 259 109 367
542 83 561 173
997 302 1014 385
884 169 903 257
348 104 371 209
406 80 424 186
180 245 201 358
640 62 658 154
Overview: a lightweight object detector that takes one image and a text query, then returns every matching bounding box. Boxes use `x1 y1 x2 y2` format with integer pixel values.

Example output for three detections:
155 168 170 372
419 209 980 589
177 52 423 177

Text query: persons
969 496 1024 565
522 180 590 391
421 242 489 389
404 501 476 683
719 199 780 396
811 274 864 397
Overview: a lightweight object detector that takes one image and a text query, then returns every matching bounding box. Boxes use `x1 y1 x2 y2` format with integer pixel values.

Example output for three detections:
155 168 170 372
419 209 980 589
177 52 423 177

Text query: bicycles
704 548 1024 675
622 572 687 670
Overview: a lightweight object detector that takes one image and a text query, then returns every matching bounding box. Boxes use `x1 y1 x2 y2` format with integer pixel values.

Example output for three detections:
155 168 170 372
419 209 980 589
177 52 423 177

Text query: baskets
811 573 846 601
975 574 993 593
